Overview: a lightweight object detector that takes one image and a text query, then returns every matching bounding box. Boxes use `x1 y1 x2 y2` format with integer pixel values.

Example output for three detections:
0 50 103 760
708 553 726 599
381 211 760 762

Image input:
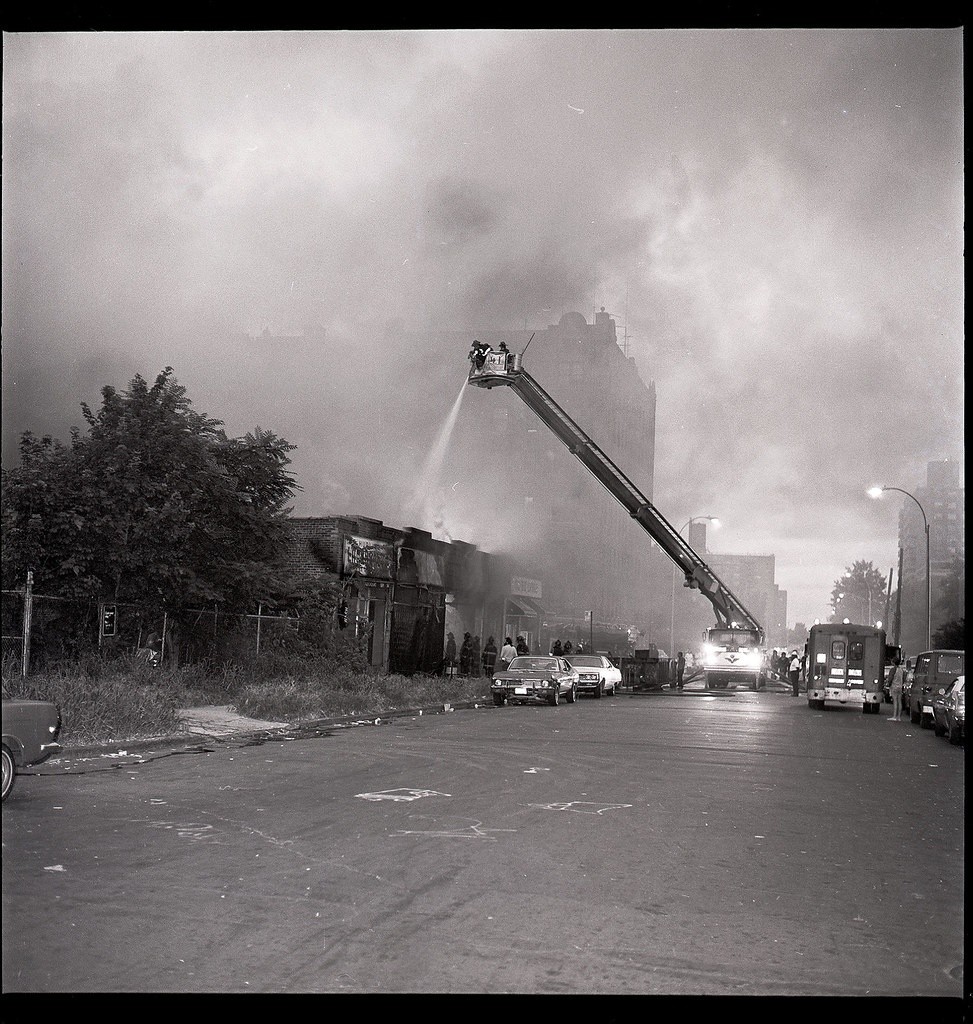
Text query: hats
791 650 797 656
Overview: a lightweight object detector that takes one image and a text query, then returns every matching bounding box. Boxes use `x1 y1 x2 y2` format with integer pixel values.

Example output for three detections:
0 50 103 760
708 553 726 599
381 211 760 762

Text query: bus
805 621 884 715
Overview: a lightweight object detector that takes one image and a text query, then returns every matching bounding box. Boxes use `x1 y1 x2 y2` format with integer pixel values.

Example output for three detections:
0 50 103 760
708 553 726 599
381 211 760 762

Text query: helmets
577 643 583 648
475 635 480 641
464 632 471 638
518 636 524 642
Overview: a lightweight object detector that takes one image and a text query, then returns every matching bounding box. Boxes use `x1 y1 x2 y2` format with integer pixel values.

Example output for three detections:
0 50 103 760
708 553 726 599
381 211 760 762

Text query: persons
770 650 789 679
886 657 902 722
460 631 481 677
563 640 573 655
516 635 530 656
445 631 456 660
677 652 685 688
481 635 497 677
499 637 518 671
790 652 800 697
551 640 564 656
575 643 583 654
903 660 912 710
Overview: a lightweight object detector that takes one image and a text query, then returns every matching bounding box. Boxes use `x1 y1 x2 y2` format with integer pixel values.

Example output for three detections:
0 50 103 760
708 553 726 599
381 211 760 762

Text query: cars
2 701 64 804
933 675 964 745
883 643 917 715
560 654 622 699
491 655 581 706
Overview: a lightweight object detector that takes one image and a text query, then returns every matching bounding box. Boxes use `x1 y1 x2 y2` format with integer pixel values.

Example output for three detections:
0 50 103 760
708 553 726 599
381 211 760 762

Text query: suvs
906 650 965 729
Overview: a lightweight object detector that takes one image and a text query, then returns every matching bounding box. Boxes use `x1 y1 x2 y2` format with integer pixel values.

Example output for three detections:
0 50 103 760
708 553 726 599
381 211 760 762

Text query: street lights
669 513 722 666
867 486 932 649
847 571 873 627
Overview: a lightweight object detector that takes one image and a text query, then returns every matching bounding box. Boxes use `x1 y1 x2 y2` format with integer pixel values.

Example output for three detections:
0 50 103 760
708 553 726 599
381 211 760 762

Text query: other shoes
886 717 901 722
791 694 798 697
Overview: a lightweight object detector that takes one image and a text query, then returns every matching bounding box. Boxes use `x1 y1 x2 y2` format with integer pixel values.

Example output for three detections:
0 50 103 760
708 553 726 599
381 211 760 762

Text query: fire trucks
467 331 808 693
